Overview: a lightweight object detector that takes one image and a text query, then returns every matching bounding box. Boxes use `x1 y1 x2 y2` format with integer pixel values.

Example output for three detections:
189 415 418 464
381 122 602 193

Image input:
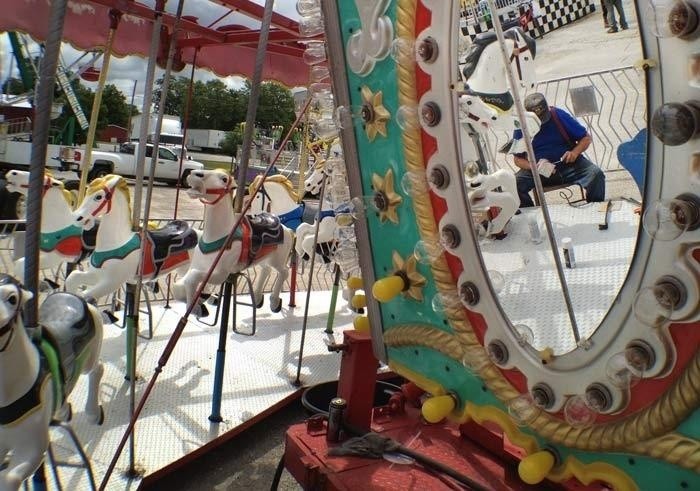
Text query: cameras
552 161 562 169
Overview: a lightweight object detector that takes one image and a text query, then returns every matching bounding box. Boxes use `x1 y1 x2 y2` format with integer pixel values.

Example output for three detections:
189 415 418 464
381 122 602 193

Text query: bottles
526 213 541 242
560 236 576 268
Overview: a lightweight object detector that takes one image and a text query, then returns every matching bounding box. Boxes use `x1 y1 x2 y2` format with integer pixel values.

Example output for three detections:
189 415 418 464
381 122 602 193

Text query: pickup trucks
52 141 204 189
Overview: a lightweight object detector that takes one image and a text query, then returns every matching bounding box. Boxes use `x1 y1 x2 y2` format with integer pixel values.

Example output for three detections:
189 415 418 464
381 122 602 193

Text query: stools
533 181 587 206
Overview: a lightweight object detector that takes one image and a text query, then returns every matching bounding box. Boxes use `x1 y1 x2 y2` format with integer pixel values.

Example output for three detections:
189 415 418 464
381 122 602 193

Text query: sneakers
605 21 628 33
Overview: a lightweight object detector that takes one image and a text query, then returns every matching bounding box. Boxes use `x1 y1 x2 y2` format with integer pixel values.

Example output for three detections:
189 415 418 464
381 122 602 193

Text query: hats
524 93 551 126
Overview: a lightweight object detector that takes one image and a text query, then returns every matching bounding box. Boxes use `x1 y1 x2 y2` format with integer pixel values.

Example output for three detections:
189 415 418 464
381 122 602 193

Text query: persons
602 0 629 33
512 92 606 208
463 159 522 240
600 0 617 28
